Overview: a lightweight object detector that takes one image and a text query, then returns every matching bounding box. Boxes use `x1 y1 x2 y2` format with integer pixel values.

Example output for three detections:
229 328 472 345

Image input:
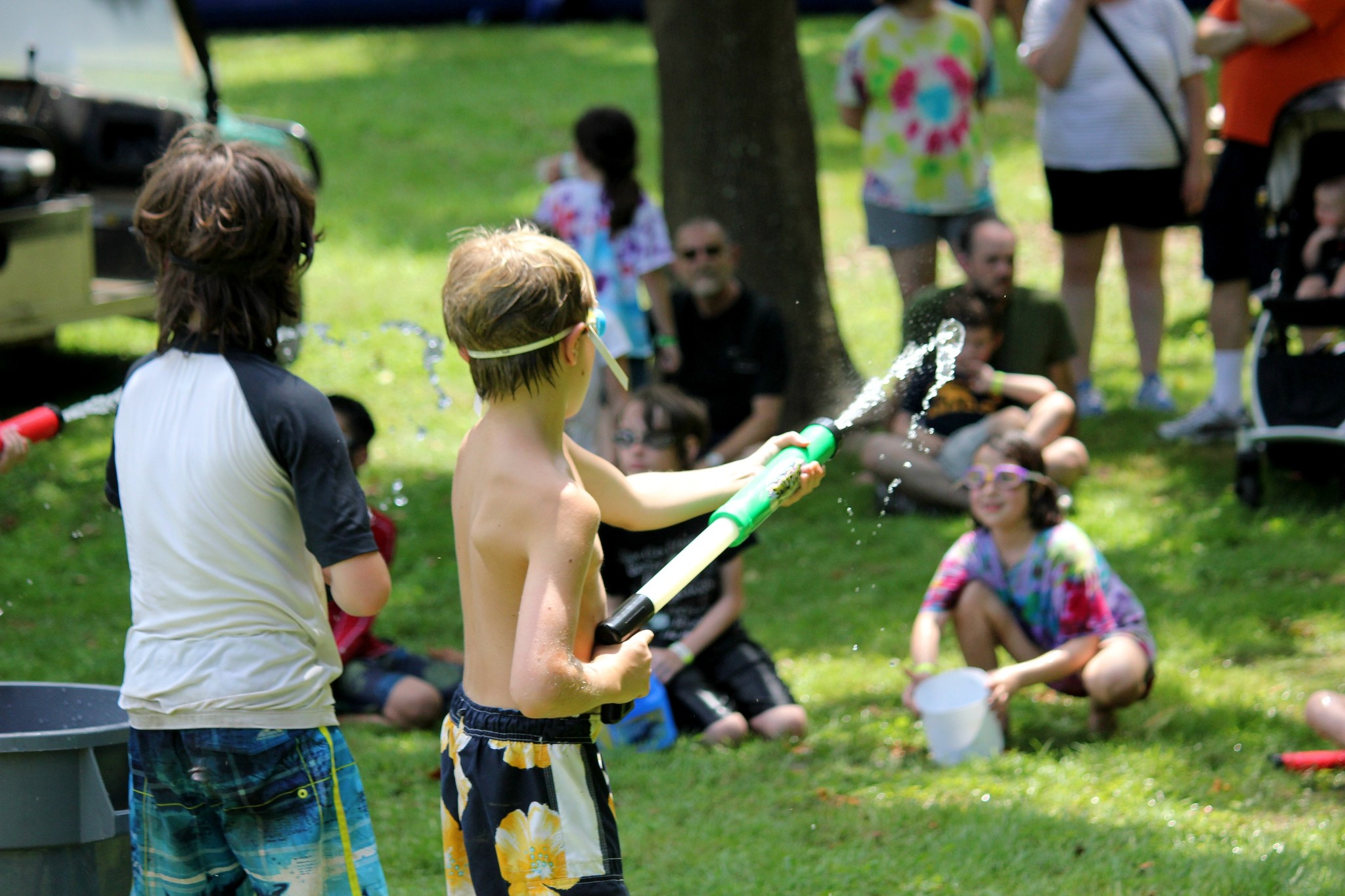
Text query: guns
0 402 66 455
592 414 842 728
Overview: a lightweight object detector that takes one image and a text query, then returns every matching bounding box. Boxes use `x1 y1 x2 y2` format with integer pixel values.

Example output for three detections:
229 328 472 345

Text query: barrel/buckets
910 664 1005 767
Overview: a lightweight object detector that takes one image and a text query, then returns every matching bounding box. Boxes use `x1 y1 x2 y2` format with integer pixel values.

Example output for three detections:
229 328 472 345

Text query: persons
102 0 1345 896
0 425 29 472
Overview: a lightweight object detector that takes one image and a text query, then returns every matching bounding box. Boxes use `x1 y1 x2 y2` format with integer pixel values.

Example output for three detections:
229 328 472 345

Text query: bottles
606 670 677 754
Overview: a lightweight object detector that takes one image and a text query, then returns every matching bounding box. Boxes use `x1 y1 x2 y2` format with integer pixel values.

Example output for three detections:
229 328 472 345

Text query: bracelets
655 333 678 347
669 641 695 666
910 663 937 675
990 370 1005 397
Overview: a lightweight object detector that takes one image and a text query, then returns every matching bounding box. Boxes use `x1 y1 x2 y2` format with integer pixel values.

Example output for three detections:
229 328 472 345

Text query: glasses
676 242 733 261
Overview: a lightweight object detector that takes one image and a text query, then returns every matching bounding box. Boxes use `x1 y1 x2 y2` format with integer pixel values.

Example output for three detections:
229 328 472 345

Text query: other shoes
1070 377 1108 416
1136 378 1173 414
874 484 917 515
1157 401 1255 446
1085 696 1116 741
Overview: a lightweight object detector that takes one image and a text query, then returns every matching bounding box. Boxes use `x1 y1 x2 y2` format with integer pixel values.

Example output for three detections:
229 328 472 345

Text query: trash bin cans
0 677 131 894
